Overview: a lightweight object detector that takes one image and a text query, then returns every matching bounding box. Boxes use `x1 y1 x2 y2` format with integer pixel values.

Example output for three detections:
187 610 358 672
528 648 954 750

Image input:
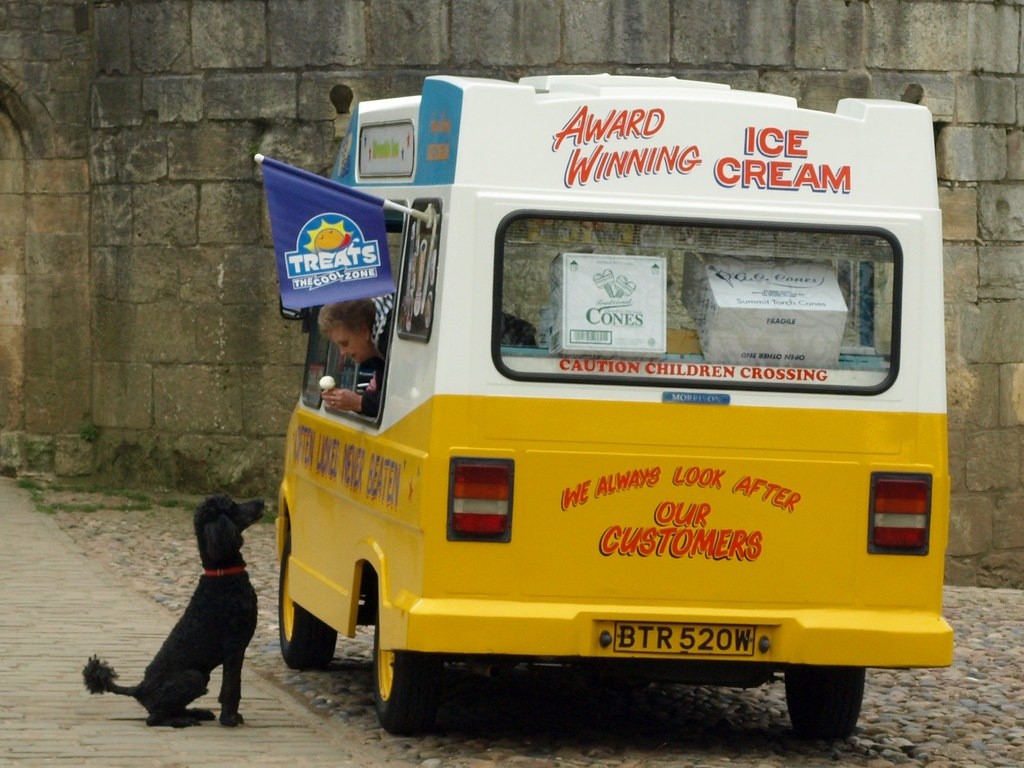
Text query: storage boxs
548 252 668 359
680 251 848 370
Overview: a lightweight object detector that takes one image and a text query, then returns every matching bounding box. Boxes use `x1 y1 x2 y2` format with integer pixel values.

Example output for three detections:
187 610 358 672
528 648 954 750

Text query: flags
253 153 397 310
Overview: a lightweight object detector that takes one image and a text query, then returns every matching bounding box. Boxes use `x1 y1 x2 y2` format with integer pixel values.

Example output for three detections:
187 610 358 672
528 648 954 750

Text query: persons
316 291 537 418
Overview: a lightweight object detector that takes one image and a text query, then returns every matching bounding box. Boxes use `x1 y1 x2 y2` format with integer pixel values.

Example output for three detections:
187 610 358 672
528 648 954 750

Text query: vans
251 71 954 746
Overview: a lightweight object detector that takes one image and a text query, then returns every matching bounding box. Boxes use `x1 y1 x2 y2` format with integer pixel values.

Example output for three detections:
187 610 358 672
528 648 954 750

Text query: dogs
83 495 265 728
